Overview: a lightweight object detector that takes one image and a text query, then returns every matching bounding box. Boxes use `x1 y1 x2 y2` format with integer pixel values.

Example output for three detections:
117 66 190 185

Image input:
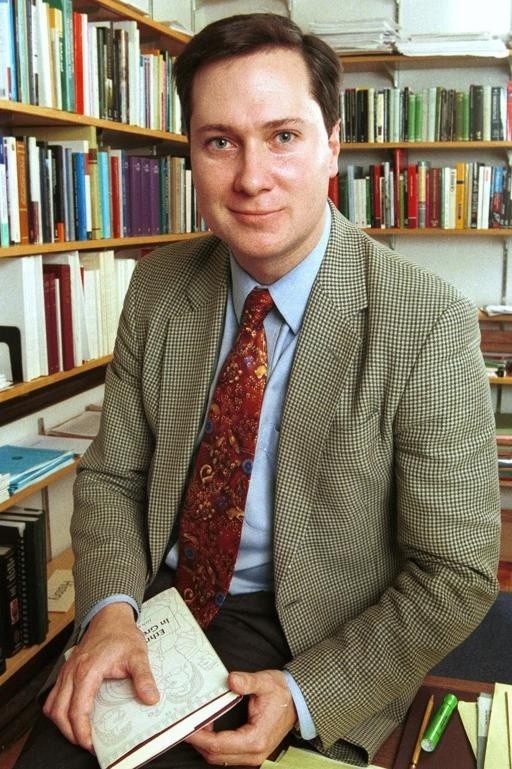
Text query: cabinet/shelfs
0 3 230 763
329 49 511 579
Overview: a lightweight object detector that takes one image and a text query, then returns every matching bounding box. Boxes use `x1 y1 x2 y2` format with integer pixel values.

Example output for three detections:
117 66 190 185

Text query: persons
13 12 501 769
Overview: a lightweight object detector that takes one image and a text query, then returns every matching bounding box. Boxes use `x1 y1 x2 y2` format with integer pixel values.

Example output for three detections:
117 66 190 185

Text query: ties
175 290 283 631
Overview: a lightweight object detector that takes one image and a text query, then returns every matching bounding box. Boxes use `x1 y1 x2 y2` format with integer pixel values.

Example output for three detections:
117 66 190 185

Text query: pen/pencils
408 694 434 769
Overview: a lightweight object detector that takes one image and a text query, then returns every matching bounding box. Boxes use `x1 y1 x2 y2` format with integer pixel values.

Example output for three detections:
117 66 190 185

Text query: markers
420 693 458 752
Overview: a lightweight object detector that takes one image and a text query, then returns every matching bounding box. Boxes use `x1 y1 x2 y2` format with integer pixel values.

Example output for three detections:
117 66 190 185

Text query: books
392 682 511 769
87 586 243 768
1 442 74 672
